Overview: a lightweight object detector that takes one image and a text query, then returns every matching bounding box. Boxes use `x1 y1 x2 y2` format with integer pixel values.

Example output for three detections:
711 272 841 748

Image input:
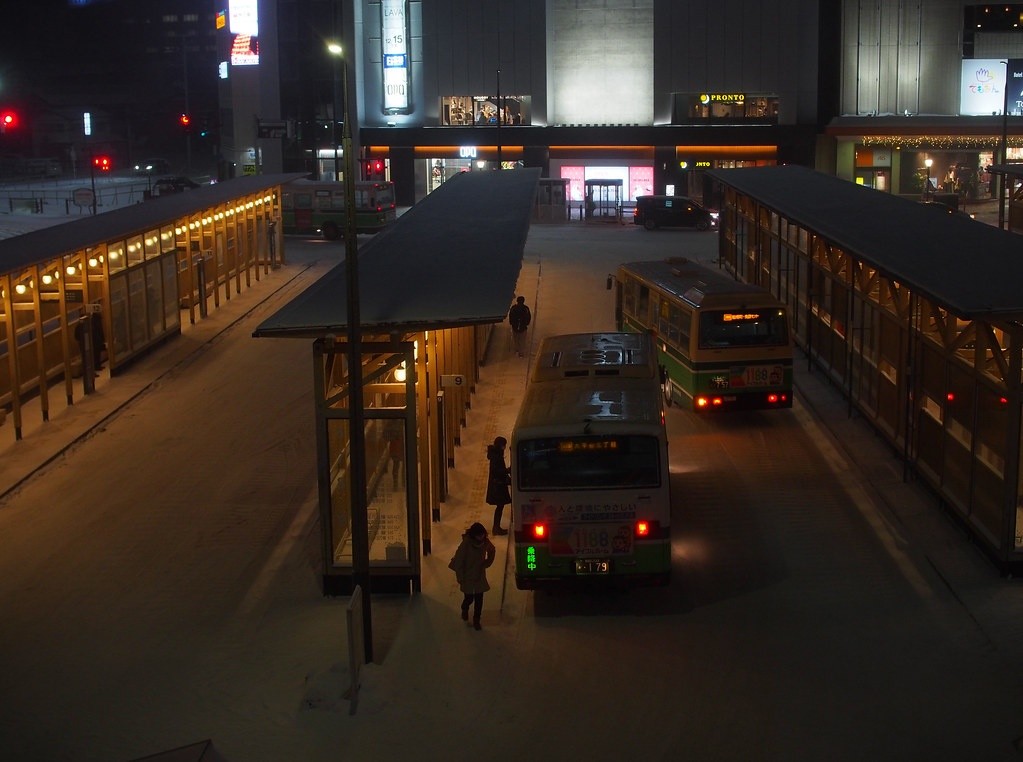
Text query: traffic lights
198 121 207 140
94 158 109 170
2 112 17 129
181 115 190 124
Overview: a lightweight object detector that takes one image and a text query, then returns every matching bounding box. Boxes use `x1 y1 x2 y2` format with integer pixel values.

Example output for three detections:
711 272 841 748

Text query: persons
509 296 531 359
74 310 106 379
456 521 497 632
479 113 497 125
485 436 512 535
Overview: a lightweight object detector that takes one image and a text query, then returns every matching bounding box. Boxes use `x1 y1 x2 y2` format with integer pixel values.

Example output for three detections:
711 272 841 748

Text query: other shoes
492 526 508 535
473 616 481 631
462 608 469 621
519 353 523 359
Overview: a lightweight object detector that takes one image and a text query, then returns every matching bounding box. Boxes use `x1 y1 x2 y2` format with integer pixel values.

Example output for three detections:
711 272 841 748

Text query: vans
634 194 713 232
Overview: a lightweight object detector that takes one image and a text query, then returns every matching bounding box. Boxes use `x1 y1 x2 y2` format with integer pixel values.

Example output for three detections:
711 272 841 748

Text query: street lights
325 42 377 664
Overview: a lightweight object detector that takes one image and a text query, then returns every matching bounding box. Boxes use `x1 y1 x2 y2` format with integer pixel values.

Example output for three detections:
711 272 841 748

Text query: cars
150 176 202 199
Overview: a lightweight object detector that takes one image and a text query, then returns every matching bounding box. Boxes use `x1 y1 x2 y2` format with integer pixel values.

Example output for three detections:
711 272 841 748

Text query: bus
256 179 397 241
509 331 673 591
606 257 794 414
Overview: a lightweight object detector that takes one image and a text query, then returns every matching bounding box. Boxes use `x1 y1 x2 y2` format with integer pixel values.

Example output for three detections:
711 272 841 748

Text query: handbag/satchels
448 558 473 576
